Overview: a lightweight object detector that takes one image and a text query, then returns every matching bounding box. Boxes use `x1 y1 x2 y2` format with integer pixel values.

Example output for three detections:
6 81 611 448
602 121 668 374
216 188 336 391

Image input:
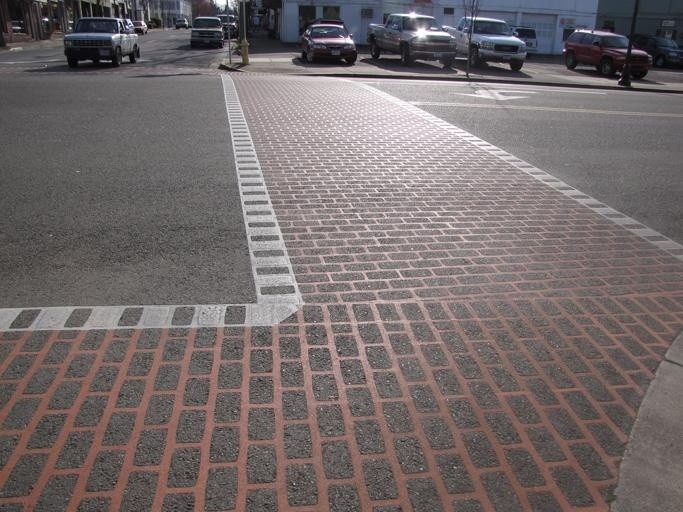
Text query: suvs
630 31 682 68
562 27 652 80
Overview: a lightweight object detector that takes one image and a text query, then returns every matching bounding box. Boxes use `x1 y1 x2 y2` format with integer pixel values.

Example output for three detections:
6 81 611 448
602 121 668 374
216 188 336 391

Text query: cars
217 14 238 38
297 23 357 64
175 18 188 28
132 20 148 34
122 19 135 31
190 17 224 48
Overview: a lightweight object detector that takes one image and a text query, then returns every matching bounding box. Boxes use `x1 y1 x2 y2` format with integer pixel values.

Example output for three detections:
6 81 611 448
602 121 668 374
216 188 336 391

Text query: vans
509 24 537 56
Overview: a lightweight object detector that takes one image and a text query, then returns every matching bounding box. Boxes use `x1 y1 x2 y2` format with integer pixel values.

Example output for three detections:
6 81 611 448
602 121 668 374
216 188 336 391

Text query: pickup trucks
365 13 457 68
61 19 140 66
441 16 527 72
